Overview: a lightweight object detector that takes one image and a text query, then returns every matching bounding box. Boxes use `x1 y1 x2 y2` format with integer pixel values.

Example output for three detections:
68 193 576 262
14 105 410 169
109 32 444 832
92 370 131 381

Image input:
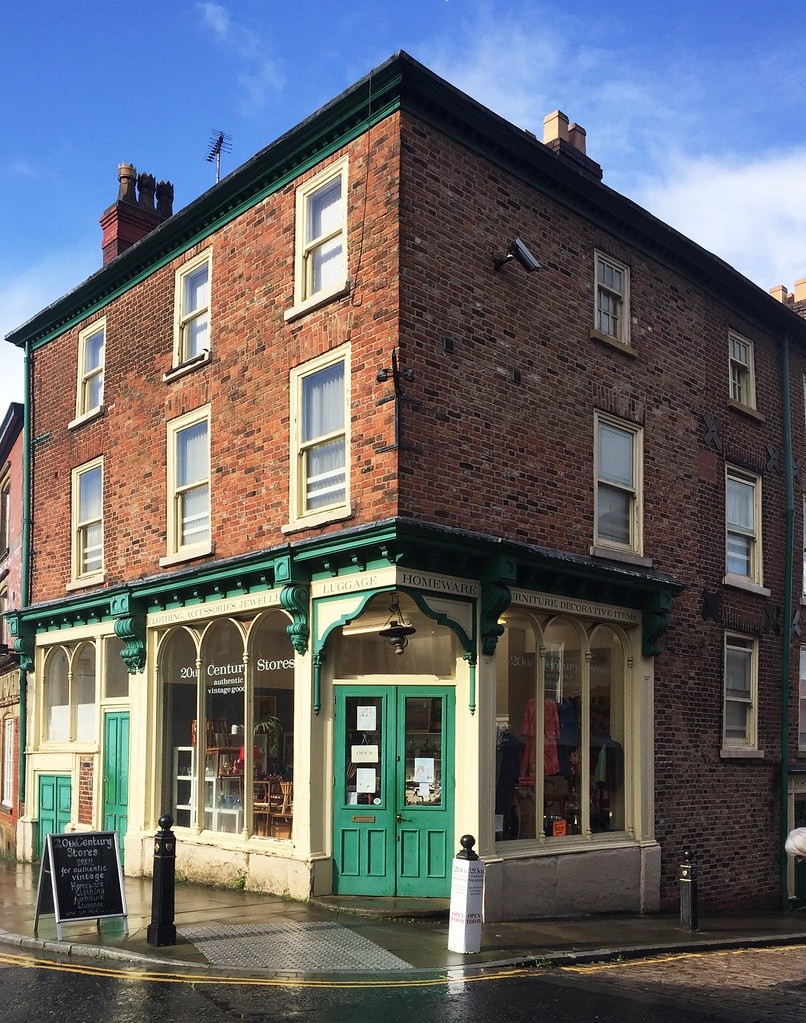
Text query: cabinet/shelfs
174 746 292 837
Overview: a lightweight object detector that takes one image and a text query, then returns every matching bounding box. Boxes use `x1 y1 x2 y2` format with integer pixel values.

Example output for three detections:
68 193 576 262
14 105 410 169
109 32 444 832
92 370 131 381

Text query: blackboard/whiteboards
36 830 128 923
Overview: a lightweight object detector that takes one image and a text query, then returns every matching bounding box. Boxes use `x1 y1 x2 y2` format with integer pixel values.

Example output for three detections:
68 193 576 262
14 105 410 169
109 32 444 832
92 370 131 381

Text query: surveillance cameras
511 238 542 272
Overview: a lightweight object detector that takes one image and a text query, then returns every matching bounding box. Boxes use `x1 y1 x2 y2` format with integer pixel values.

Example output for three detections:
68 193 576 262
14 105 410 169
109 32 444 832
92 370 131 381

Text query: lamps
377 367 390 383
400 368 414 381
378 595 416 653
